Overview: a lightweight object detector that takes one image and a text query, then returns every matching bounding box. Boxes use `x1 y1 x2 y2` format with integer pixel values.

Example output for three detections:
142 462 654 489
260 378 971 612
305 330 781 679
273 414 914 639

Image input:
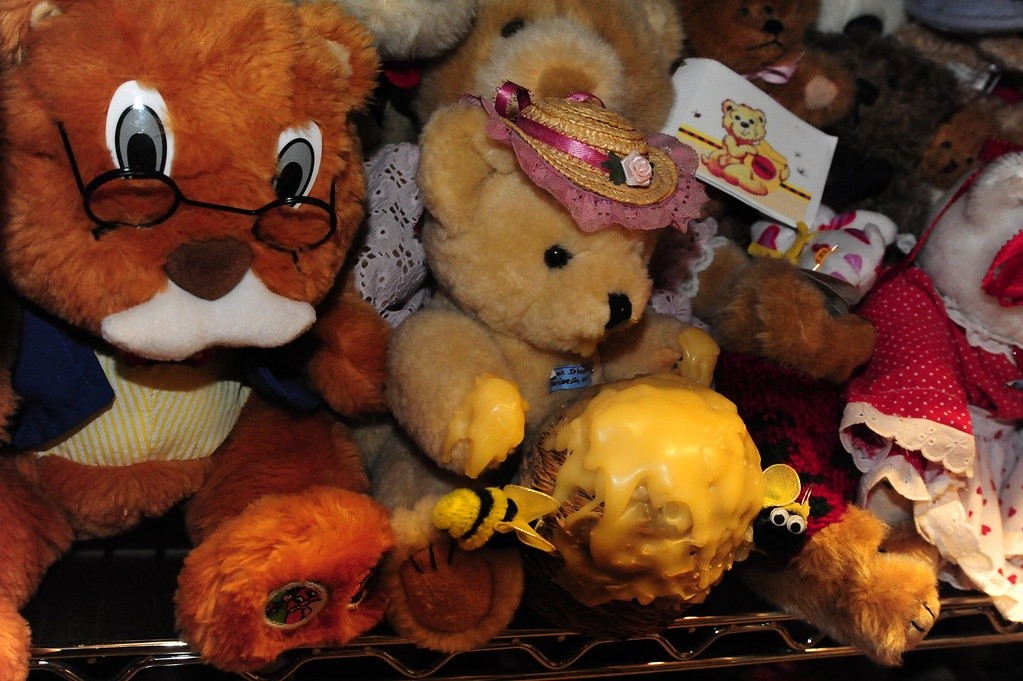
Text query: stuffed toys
340 0 1023 662
0 0 393 681
